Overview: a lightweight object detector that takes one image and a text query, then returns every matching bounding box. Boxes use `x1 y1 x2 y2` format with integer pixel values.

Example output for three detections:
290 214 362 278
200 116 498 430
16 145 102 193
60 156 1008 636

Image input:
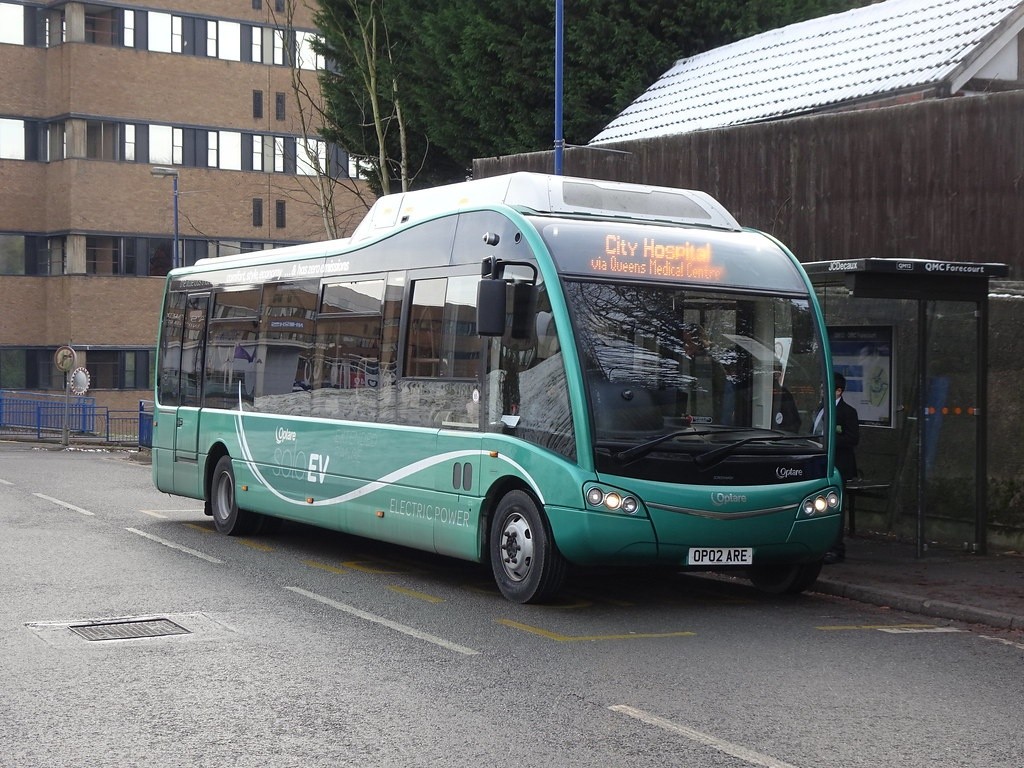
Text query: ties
814 413 825 436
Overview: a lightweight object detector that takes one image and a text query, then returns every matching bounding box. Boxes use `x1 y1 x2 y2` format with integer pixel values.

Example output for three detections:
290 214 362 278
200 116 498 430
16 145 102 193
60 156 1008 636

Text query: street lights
149 166 180 268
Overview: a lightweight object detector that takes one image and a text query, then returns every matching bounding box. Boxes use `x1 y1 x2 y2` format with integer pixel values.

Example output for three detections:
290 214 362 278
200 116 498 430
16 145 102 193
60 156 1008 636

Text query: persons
678 321 728 424
771 357 803 434
808 372 860 565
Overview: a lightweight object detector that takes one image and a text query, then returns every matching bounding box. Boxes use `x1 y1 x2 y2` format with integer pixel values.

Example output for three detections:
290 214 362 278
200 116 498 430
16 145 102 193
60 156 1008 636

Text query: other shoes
822 548 845 565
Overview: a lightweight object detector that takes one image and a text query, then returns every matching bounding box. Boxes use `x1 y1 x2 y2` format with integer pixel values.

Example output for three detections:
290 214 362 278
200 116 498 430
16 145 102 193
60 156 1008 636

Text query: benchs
844 479 896 537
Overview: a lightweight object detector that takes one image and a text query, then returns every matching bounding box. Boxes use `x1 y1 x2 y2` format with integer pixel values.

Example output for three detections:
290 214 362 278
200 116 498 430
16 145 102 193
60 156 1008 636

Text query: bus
152 173 845 603
